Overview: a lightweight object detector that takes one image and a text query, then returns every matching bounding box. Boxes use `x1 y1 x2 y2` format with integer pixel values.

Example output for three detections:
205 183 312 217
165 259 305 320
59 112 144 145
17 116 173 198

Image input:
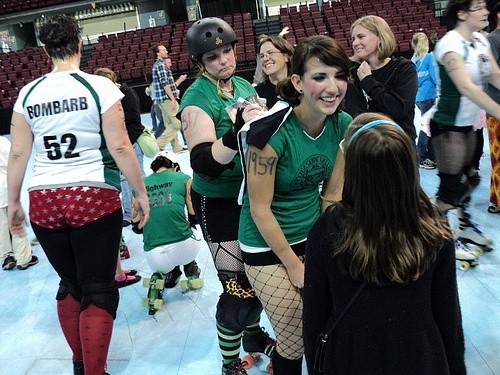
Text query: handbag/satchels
136 125 159 158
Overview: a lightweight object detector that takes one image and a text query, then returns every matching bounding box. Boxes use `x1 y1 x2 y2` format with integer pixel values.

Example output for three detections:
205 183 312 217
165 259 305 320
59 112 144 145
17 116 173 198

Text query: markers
237 96 252 107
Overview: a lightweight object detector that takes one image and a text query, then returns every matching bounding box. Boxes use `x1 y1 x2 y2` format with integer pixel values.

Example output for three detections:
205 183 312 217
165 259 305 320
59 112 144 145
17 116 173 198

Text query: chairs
83 12 255 83
0 45 55 110
278 0 448 57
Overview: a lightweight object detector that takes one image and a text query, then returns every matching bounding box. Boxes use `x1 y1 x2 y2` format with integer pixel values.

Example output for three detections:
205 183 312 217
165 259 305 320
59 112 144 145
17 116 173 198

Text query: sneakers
488 202 500 213
419 158 437 169
17 256 38 270
2 255 15 270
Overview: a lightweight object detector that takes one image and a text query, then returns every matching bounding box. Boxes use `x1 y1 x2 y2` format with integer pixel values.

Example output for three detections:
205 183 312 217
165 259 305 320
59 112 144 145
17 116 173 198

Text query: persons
251 25 290 89
113 246 142 287
302 111 467 374
408 30 436 171
238 33 351 375
431 0 499 269
6 16 150 375
30 237 41 245
131 149 205 316
0 136 38 271
173 18 279 375
340 15 418 148
147 41 188 155
94 67 148 233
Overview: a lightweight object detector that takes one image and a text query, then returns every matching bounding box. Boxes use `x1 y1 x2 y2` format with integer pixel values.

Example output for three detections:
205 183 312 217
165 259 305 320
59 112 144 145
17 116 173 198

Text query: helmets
186 17 238 64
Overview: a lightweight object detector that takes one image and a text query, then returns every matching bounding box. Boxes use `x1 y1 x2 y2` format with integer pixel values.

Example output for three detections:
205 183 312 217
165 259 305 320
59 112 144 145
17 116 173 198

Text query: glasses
259 51 283 59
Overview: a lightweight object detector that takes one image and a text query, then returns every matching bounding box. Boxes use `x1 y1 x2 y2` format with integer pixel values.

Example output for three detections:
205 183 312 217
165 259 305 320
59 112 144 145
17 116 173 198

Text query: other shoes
118 245 130 260
115 274 142 288
124 270 137 275
175 148 188 155
121 220 130 227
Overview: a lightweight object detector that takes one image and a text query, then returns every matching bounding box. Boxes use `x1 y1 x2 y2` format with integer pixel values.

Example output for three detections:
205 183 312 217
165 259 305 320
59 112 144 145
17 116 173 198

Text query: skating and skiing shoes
222 358 248 375
141 273 164 314
241 327 276 375
454 216 494 252
179 262 202 294
453 238 479 271
